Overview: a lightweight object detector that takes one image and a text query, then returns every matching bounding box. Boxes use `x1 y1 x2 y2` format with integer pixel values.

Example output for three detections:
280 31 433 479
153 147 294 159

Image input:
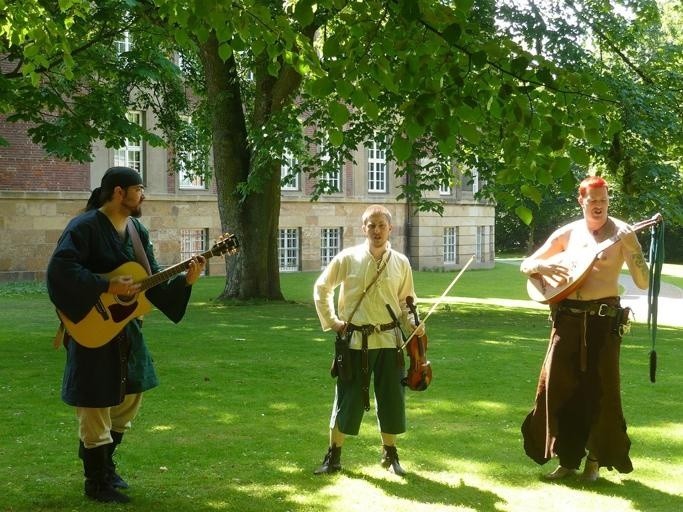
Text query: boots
381 444 406 478
79 427 135 504
313 443 341 476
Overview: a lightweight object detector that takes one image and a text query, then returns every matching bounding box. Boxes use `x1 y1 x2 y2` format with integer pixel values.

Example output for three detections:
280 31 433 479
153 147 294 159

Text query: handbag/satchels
330 245 393 379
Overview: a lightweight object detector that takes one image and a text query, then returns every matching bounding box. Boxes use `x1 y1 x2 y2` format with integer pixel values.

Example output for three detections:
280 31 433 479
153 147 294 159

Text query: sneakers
578 457 600 483
544 464 575 480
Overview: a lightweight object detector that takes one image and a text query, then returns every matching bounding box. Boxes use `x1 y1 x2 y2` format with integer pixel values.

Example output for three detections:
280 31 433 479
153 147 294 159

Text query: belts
558 303 618 375
346 321 400 412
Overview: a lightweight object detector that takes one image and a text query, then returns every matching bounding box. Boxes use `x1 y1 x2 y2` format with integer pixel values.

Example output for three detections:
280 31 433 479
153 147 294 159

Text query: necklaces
592 217 608 235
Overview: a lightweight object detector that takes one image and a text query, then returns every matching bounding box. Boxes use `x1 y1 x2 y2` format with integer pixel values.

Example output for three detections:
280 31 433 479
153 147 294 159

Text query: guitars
56 233 241 350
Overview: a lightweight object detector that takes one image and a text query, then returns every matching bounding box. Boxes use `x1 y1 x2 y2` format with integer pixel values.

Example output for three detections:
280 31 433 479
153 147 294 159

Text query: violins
406 296 431 391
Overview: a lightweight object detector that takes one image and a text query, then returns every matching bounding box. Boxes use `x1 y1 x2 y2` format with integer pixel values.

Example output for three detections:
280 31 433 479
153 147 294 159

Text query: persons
314 205 425 475
520 176 651 484
46 167 206 503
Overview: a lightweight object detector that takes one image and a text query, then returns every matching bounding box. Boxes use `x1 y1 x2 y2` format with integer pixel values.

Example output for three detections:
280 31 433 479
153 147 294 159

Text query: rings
127 290 131 296
